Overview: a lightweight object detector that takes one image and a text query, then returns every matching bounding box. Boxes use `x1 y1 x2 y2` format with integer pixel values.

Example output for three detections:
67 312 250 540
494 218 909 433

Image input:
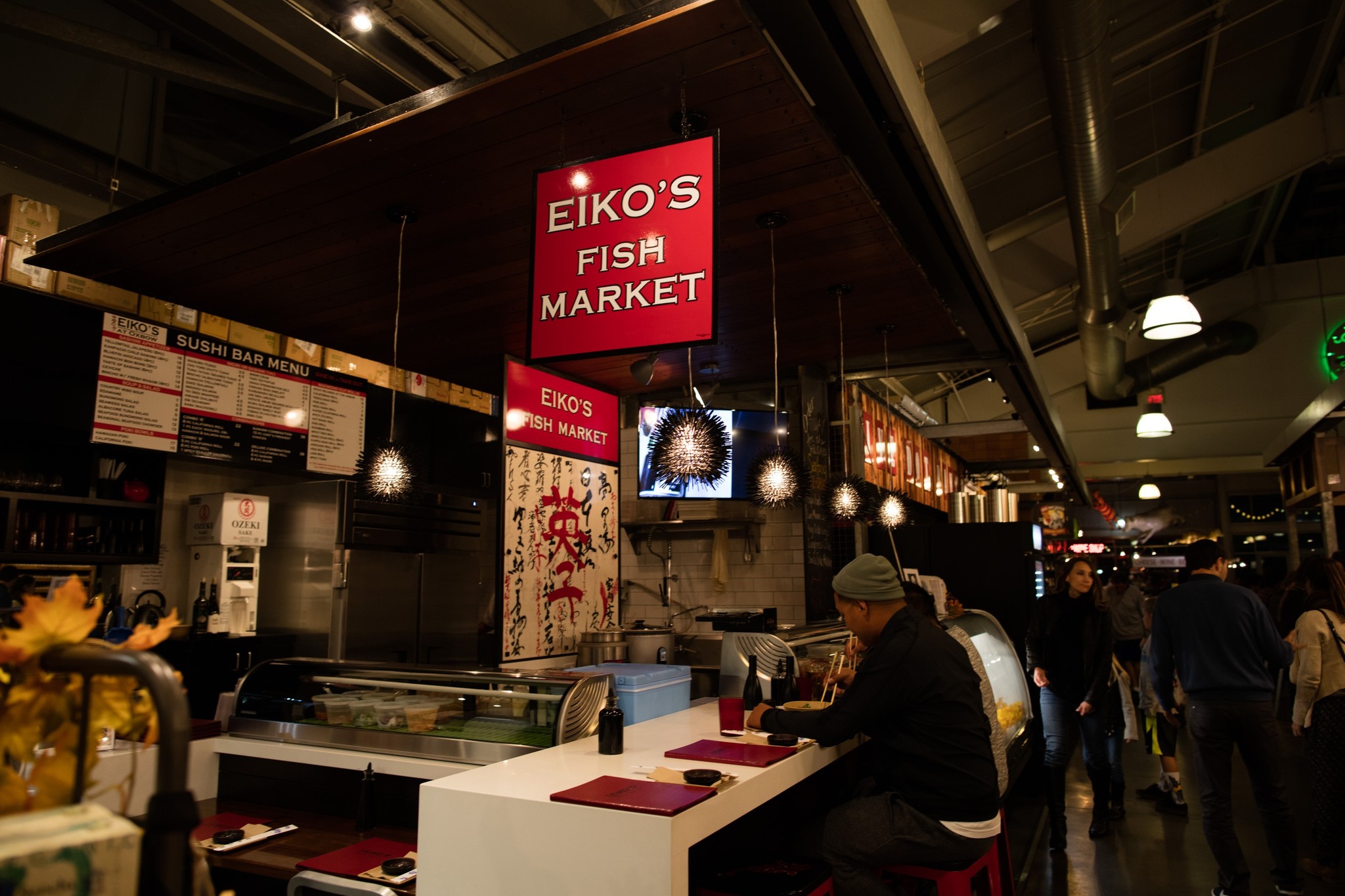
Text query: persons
1025 556 1113 849
1101 537 1345 896
746 554 1008 896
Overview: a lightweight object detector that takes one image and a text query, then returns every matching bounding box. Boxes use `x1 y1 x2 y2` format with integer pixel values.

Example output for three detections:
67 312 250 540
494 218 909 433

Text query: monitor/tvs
637 406 791 501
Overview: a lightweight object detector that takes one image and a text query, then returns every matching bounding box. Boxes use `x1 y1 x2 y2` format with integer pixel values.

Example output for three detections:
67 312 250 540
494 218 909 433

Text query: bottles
742 655 763 711
192 577 209 635
771 656 800 708
206 577 220 636
598 696 624 755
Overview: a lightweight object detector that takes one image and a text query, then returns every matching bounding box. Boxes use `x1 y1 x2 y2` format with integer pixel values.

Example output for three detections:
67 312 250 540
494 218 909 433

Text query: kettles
125 589 166 630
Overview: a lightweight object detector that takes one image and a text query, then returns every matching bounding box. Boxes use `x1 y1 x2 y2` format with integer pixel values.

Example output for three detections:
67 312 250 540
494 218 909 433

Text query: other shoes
1131 689 1140 706
1211 887 1232 896
1154 796 1188 816
1273 877 1304 895
1135 782 1173 800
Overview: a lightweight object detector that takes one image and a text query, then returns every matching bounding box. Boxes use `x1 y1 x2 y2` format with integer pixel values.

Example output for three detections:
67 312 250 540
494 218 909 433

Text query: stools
876 806 1004 896
694 856 846 896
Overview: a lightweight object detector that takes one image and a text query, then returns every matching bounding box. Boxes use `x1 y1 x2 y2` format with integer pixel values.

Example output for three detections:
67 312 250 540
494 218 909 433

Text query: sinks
668 633 722 668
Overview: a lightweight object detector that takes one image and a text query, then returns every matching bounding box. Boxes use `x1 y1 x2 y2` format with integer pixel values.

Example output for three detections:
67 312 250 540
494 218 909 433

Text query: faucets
667 604 711 627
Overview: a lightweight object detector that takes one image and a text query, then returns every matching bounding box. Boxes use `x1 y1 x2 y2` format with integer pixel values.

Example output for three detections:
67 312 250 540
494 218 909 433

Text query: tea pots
122 478 150 503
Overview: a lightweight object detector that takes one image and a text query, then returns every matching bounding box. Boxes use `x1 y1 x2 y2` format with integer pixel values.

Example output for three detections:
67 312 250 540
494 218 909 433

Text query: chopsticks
819 652 845 709
849 632 858 671
99 458 126 478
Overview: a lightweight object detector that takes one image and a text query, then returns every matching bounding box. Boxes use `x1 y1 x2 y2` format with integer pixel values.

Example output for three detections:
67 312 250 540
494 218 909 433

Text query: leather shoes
1300 858 1340 883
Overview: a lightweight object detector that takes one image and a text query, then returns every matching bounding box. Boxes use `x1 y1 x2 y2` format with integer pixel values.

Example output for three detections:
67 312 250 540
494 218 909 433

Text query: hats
831 553 905 602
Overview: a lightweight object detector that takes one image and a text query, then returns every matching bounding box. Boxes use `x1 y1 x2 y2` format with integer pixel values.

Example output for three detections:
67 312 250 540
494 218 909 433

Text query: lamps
1132 355 1177 442
649 347 727 489
751 211 806 510
824 290 866 523
876 323 909 529
352 217 420 501
1136 468 1164 502
1136 68 1205 344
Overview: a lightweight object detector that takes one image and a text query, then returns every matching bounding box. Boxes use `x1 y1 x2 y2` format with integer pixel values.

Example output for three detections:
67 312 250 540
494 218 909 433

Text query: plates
381 857 416 876
683 769 721 786
213 829 245 844
767 734 798 747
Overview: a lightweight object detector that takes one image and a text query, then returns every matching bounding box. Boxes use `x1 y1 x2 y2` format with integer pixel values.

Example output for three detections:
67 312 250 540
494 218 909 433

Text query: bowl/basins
783 701 831 711
373 701 410 729
348 699 384 726
403 704 440 732
312 694 347 721
342 690 455 725
323 697 359 725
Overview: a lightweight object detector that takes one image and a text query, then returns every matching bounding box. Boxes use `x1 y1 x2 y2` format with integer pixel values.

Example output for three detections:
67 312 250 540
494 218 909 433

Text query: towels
710 526 730 592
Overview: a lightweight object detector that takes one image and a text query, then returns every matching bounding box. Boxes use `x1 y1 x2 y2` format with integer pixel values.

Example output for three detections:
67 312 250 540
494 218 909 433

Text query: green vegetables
802 703 811 709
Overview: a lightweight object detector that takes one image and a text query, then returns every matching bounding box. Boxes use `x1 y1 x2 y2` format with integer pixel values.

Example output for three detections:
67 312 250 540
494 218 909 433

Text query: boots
1045 767 1068 850
1108 781 1126 822
1087 765 1111 838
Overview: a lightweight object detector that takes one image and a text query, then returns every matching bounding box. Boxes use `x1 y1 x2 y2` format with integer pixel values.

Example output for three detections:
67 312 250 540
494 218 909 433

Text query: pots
575 631 631 667
605 620 676 665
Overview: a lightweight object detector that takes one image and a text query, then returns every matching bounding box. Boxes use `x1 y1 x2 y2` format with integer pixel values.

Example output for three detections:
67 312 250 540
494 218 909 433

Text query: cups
97 478 117 500
718 698 745 737
805 665 820 684
